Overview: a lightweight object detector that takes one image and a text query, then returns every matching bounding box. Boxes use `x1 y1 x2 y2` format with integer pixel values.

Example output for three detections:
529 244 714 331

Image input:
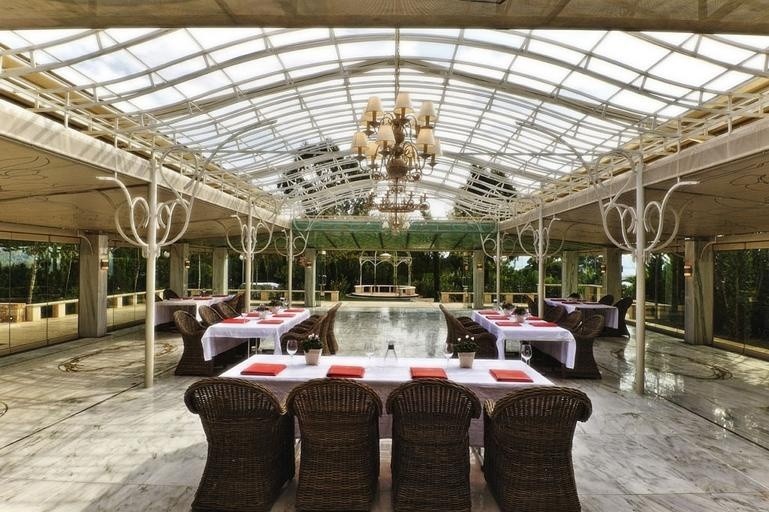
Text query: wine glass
240 296 290 326
520 344 532 374
524 308 533 320
284 340 300 370
362 341 377 368
442 342 455 373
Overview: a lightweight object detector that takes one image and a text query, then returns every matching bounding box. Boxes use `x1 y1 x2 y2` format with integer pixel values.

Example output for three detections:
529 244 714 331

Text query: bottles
181 288 214 300
492 299 500 313
383 339 398 371
565 291 585 303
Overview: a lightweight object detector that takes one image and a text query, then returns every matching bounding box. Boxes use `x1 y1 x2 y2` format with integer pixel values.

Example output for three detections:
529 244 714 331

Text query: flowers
452 333 481 356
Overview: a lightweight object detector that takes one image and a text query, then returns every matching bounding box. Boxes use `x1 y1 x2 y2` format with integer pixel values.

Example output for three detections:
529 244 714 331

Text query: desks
217 353 557 473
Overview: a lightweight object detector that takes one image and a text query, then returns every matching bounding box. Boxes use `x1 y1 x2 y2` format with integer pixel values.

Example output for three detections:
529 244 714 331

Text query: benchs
26 288 167 323
354 284 417 297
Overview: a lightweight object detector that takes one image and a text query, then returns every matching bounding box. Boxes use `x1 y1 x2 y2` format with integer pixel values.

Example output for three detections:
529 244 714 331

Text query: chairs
144 287 343 378
183 376 296 512
438 291 634 381
482 384 593 512
284 377 384 511
384 375 482 511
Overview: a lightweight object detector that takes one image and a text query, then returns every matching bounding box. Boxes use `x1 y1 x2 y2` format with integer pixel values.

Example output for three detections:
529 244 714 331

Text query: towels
409 365 448 382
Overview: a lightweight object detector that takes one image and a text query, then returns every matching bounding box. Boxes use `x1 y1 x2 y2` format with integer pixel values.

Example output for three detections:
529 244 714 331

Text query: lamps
683 265 693 277
101 259 109 270
600 264 606 273
306 261 313 269
351 90 443 183
477 263 483 272
361 179 431 237
185 259 191 269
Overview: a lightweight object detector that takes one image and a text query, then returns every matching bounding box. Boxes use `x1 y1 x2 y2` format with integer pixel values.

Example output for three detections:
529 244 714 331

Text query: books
241 363 287 376
219 307 305 324
489 367 532 382
480 307 558 328
550 297 610 305
170 293 228 300
409 365 446 381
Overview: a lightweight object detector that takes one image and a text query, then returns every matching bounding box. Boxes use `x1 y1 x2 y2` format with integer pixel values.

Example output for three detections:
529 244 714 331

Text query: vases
457 351 476 368
